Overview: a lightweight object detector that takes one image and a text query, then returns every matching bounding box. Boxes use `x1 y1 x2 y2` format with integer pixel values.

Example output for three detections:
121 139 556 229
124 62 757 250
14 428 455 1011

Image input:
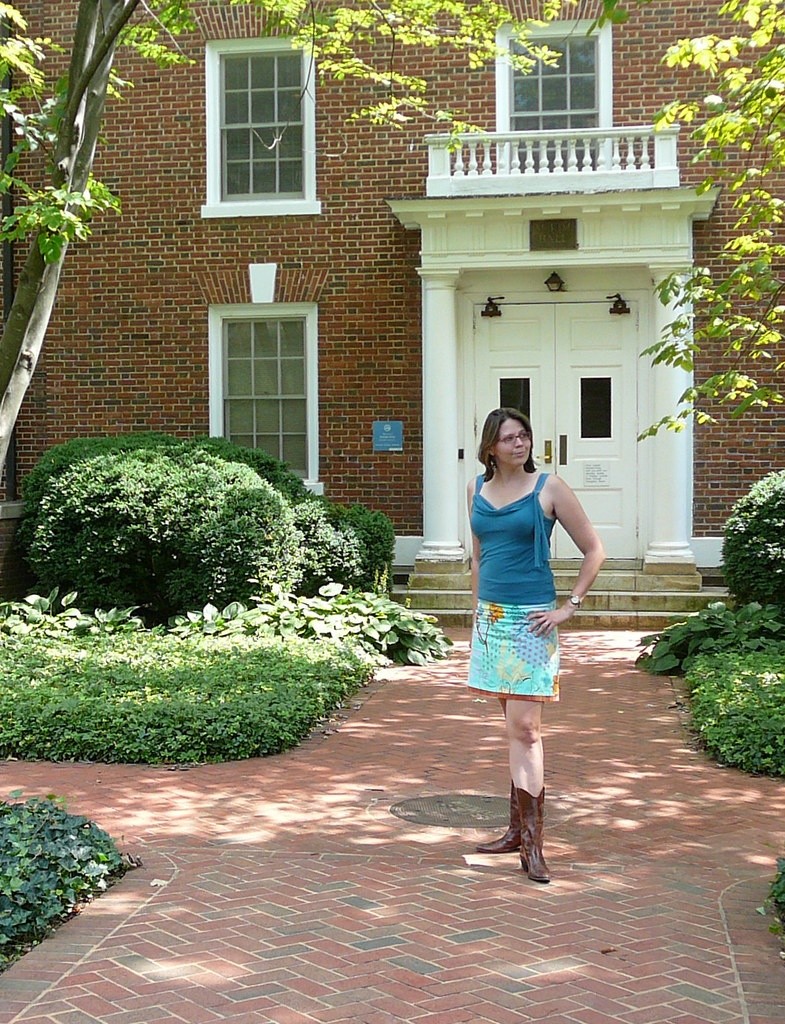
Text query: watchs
566 595 581 608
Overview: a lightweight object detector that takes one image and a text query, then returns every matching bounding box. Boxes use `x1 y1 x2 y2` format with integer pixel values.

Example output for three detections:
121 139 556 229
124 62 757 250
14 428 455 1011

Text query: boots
516 787 550 881
475 779 523 853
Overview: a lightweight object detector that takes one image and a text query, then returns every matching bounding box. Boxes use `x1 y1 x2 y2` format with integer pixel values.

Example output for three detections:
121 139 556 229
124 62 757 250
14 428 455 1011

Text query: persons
468 409 607 881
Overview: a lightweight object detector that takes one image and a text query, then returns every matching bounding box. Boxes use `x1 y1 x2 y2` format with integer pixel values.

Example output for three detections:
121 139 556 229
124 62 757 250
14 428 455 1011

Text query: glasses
496 432 532 445
